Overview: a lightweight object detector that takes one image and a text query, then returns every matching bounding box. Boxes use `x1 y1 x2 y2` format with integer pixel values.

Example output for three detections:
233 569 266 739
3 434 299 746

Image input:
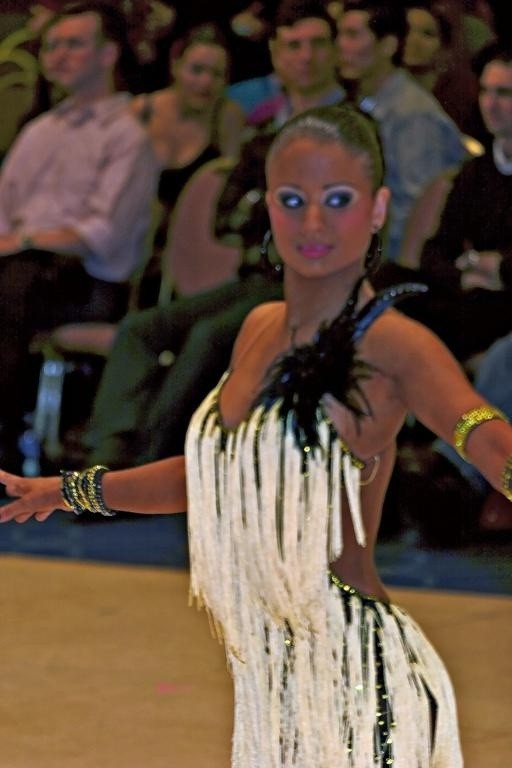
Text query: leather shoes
480 488 512 533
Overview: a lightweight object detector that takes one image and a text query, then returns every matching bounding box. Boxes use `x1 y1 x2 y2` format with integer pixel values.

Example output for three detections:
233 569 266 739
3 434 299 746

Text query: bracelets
501 459 512 503
60 464 118 521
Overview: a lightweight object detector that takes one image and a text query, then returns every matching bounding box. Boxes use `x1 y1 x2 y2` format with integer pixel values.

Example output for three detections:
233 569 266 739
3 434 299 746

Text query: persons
0 104 512 766
2 0 512 557
